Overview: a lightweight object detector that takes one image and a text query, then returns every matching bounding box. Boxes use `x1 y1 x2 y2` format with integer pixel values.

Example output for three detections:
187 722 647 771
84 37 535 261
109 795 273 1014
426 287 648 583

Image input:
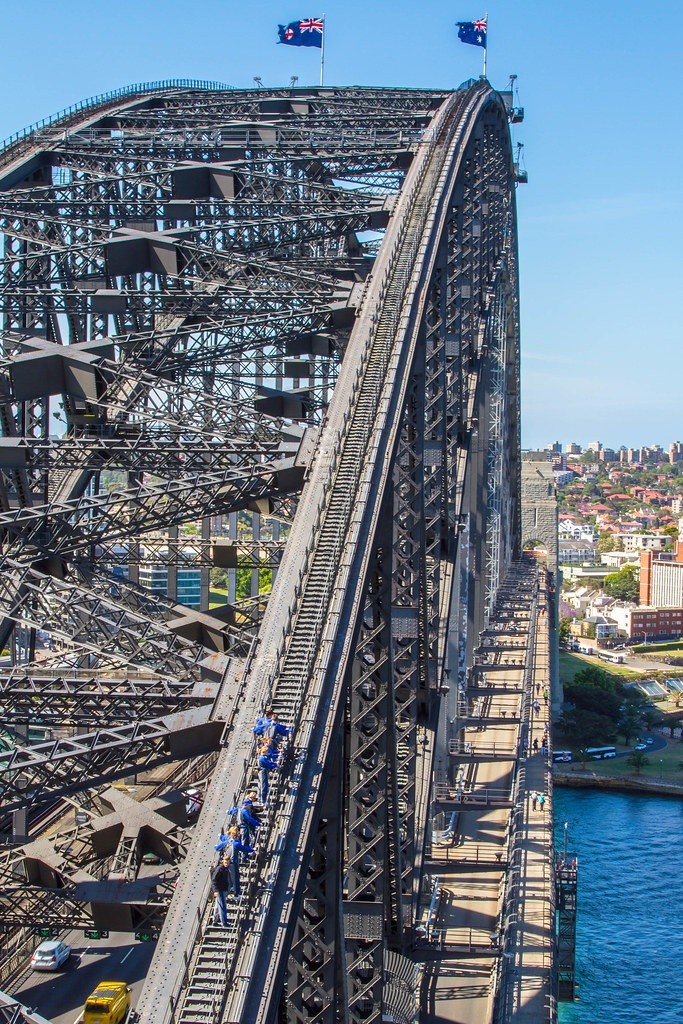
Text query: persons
636 737 641 743
234 792 267 864
219 826 258 899
536 683 540 695
543 690 548 703
538 793 545 810
213 856 232 927
534 699 540 718
533 738 538 753
541 680 545 692
531 791 538 811
254 709 292 807
544 724 548 733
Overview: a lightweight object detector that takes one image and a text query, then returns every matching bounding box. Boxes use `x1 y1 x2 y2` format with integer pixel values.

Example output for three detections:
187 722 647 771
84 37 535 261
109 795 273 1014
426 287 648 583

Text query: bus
553 750 572 763
580 747 616 759
598 650 623 663
84 981 132 1024
573 643 592 655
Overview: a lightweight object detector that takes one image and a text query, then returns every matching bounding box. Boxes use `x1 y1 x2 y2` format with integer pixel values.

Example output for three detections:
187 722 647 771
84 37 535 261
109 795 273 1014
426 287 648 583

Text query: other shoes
223 923 231 926
213 922 221 926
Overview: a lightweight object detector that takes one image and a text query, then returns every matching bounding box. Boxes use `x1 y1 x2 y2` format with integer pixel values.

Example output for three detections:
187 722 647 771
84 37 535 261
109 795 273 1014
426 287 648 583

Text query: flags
457 18 486 49
277 17 323 49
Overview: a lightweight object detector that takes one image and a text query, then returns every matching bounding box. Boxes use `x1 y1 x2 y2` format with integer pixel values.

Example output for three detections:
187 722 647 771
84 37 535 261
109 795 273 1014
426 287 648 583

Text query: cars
647 738 653 744
30 940 70 971
635 744 646 750
